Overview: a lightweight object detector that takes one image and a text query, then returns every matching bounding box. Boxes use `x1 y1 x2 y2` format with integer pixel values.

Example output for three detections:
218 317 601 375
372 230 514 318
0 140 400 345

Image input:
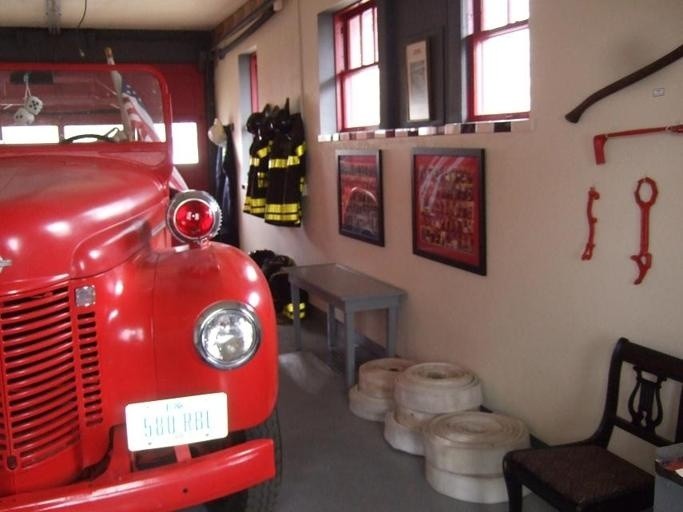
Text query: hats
207 118 229 149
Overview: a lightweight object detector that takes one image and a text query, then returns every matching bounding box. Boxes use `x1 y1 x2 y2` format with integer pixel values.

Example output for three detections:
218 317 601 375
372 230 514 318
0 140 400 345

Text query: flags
109 70 189 193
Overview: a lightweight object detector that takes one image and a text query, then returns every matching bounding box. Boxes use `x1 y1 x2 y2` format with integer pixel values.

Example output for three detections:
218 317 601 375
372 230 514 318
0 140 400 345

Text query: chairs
496 334 682 512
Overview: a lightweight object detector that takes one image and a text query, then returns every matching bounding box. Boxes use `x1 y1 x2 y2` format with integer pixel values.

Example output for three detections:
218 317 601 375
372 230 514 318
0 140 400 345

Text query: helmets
246 109 292 141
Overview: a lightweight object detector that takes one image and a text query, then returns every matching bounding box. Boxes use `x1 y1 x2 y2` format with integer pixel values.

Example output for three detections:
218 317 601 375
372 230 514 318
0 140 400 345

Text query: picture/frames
405 145 490 278
332 146 388 250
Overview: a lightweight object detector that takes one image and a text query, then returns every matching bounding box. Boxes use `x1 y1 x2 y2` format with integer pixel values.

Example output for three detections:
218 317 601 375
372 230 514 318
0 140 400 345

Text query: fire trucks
0 60 282 510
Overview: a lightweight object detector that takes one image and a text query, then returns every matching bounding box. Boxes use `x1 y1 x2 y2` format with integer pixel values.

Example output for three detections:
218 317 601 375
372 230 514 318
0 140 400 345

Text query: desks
280 261 409 392
648 440 682 511
